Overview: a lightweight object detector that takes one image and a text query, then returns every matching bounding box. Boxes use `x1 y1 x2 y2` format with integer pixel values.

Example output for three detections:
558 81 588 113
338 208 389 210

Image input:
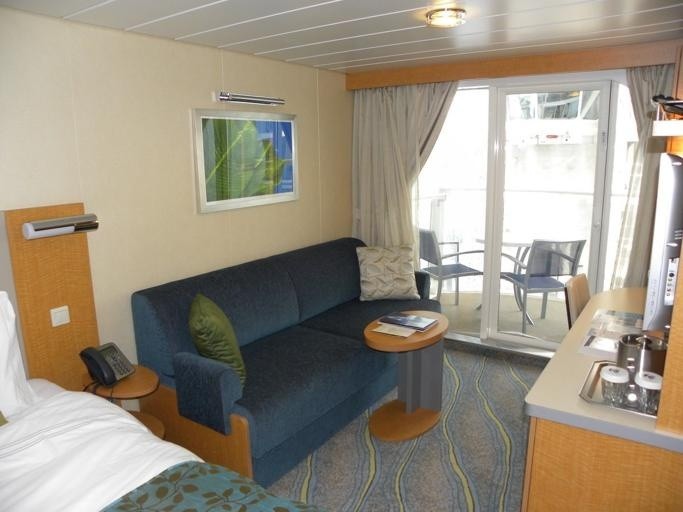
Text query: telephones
80 342 135 388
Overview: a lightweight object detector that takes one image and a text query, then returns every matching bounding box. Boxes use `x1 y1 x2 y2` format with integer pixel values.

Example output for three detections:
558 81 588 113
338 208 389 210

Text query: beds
0 202 334 512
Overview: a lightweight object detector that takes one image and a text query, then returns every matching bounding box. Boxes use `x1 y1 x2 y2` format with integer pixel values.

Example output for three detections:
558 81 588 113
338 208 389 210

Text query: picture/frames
188 107 301 214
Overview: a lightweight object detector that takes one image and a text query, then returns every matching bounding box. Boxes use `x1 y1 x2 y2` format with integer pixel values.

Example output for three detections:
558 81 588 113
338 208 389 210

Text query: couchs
131 237 441 490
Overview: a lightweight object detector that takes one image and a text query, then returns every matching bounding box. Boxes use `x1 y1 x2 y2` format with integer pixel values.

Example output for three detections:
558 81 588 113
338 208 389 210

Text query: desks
520 238 683 512
82 365 165 439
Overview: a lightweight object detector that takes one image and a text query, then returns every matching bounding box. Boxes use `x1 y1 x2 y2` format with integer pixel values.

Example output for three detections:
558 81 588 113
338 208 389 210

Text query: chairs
420 229 591 337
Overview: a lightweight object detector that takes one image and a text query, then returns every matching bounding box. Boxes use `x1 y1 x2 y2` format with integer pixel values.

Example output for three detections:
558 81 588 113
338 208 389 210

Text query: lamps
652 119 683 136
425 8 467 28
212 90 289 106
22 213 100 240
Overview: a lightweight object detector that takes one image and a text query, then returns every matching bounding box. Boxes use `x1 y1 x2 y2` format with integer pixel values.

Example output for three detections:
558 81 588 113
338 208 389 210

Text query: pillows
0 291 43 428
189 292 246 391
356 243 421 302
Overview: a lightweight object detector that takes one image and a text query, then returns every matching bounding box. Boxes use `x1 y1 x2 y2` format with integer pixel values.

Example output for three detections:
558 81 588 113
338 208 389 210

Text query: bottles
600 365 630 408
634 371 663 415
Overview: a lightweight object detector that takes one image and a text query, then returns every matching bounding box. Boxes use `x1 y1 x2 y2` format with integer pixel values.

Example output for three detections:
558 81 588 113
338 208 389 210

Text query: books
375 310 437 333
